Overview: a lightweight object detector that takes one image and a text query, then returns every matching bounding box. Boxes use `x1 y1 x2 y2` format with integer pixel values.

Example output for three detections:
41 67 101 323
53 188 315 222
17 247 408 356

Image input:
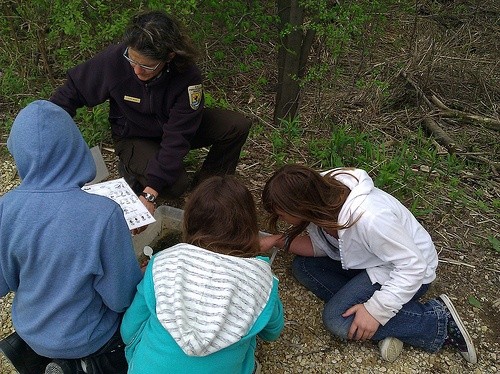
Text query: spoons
143 245 153 259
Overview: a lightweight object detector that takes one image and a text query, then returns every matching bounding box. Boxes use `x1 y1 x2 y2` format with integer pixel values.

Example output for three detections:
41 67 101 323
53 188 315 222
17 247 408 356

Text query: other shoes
378 335 403 362
432 294 478 365
45 359 75 374
0 333 45 374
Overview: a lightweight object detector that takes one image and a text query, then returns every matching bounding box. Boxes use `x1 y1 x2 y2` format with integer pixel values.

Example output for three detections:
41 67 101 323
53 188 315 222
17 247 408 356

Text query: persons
0 99 145 374
50 10 251 237
260 163 478 364
120 174 285 374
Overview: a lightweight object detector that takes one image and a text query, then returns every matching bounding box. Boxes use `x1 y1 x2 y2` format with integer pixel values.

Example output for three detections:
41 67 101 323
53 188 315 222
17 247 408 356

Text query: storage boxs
132 204 278 265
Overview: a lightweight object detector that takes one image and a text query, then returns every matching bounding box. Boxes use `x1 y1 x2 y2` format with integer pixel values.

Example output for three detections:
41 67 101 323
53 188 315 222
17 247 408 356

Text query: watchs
139 191 157 204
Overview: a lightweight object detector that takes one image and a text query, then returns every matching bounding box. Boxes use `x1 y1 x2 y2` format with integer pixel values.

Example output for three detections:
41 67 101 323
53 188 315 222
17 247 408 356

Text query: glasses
122 47 166 71
